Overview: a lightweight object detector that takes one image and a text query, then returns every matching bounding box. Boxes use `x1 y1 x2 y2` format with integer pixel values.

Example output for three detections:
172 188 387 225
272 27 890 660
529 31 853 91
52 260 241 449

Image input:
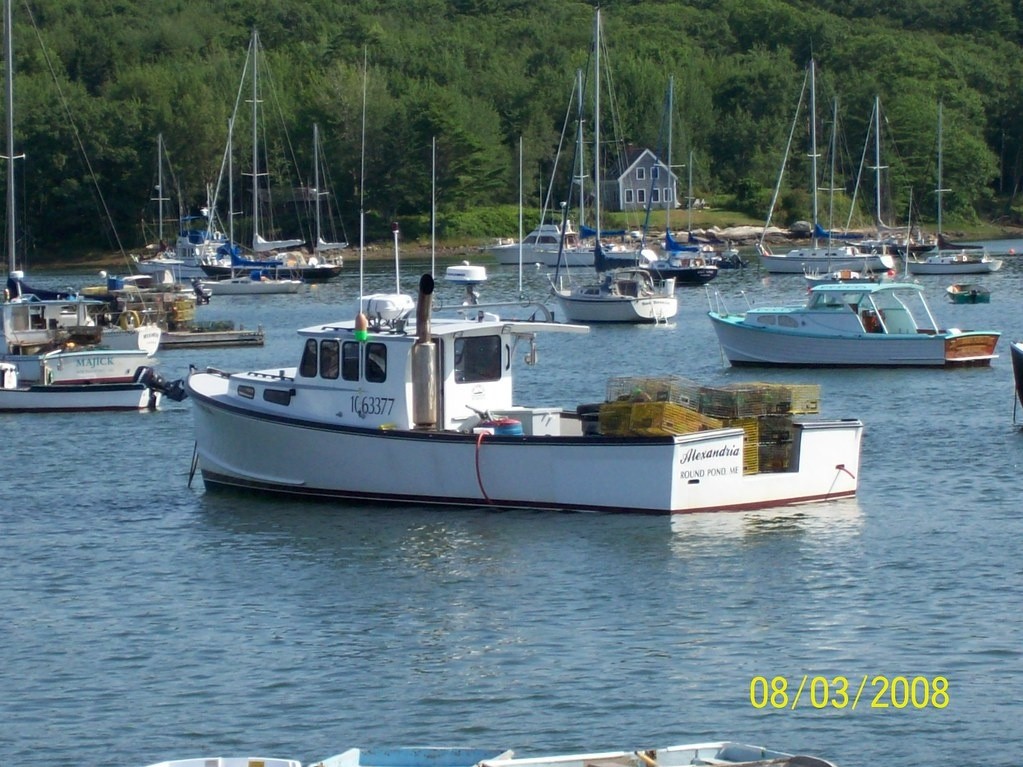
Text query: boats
1007 339 1023 428
945 281 993 304
181 44 868 515
701 182 1007 370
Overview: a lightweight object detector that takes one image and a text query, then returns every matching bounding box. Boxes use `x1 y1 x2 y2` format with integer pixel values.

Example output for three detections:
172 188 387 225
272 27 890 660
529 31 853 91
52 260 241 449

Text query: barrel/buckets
499 420 523 436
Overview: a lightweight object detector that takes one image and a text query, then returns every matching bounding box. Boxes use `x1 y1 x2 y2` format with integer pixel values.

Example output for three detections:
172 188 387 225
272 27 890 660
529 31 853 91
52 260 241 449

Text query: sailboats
534 0 679 323
752 37 897 277
893 101 1005 278
842 95 938 259
799 96 897 304
472 68 750 284
0 0 362 412
